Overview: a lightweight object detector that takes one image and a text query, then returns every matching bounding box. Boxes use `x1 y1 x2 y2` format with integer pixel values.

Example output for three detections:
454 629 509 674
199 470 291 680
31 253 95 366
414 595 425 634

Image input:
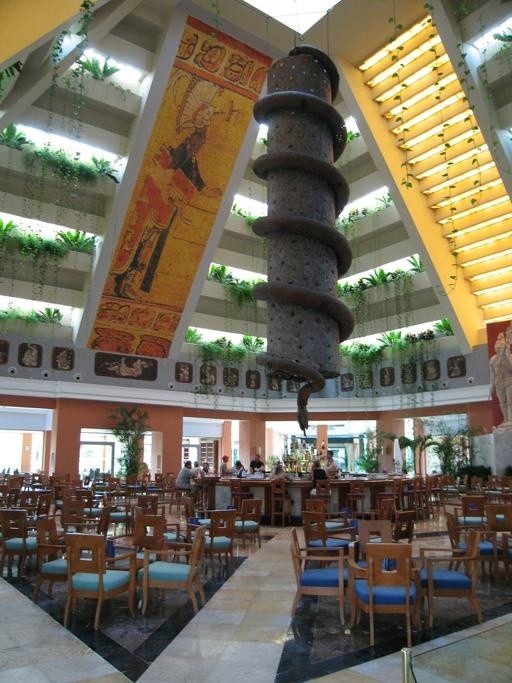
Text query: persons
323 452 337 477
309 459 326 491
220 456 229 476
192 461 200 483
176 462 196 500
201 463 211 477
233 462 246 477
250 455 264 475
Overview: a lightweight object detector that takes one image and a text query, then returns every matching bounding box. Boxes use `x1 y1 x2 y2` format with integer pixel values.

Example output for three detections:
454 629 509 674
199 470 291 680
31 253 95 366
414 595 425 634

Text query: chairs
289 525 349 625
53 484 65 526
32 517 109 603
348 518 409 568
481 503 511 532
184 502 212 539
15 494 52 526
402 476 426 522
137 524 211 615
236 498 264 552
0 508 44 584
271 478 292 527
310 479 331 518
419 530 482 628
442 510 503 584
206 507 237 576
300 510 352 569
62 532 138 629
169 477 192 514
230 478 254 513
1 472 175 506
346 479 366 519
74 489 103 531
102 493 132 541
345 543 422 647
376 478 403 525
454 495 487 525
424 471 511 517
